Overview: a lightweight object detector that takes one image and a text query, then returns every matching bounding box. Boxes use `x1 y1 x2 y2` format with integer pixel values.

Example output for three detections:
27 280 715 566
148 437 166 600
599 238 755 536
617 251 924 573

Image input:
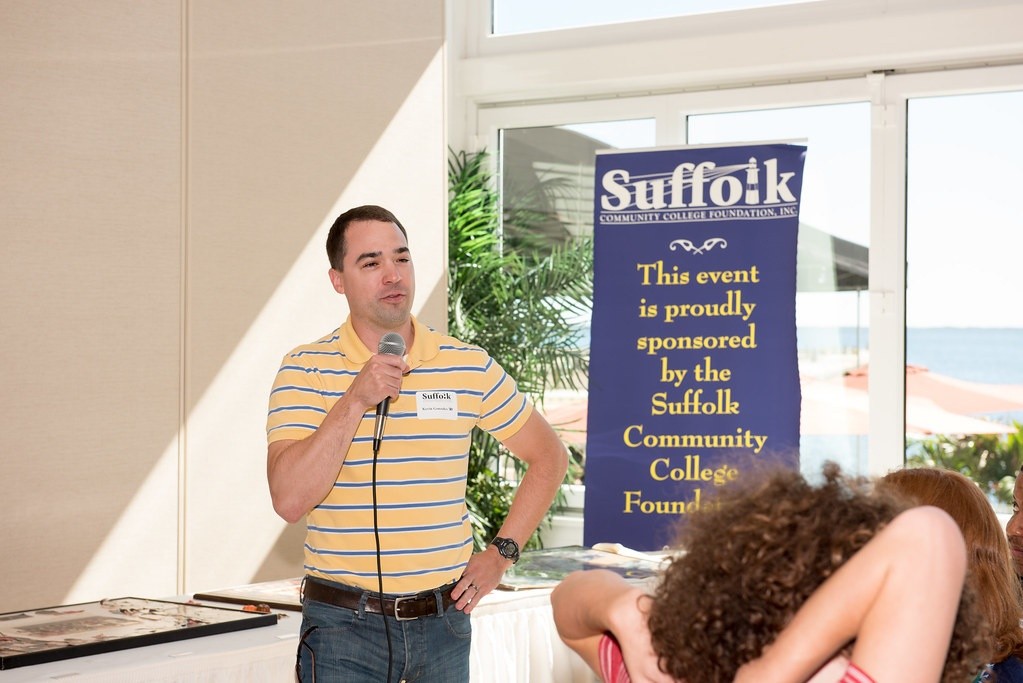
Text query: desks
0 548 693 682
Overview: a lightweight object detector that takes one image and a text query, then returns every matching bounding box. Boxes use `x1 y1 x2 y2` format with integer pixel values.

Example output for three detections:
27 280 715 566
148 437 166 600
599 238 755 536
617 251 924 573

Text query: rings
468 584 477 591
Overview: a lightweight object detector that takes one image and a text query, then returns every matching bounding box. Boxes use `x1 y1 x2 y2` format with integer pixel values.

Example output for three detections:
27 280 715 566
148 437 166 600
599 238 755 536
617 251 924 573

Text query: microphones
373 332 406 451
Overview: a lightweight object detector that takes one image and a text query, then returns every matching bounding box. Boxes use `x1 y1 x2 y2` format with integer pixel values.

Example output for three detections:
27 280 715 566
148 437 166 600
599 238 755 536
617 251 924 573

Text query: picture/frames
494 544 669 591
194 577 303 612
0 596 278 673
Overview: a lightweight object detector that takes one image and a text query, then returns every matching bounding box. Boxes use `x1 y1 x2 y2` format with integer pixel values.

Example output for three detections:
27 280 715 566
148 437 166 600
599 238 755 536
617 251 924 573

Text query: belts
303 580 464 621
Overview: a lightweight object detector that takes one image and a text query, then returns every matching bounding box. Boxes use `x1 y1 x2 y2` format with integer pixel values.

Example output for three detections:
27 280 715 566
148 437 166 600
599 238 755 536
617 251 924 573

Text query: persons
549 464 1023 683
266 206 568 683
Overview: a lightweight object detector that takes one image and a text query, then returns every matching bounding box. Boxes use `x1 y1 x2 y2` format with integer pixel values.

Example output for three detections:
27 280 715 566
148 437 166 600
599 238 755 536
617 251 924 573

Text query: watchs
491 537 520 564
